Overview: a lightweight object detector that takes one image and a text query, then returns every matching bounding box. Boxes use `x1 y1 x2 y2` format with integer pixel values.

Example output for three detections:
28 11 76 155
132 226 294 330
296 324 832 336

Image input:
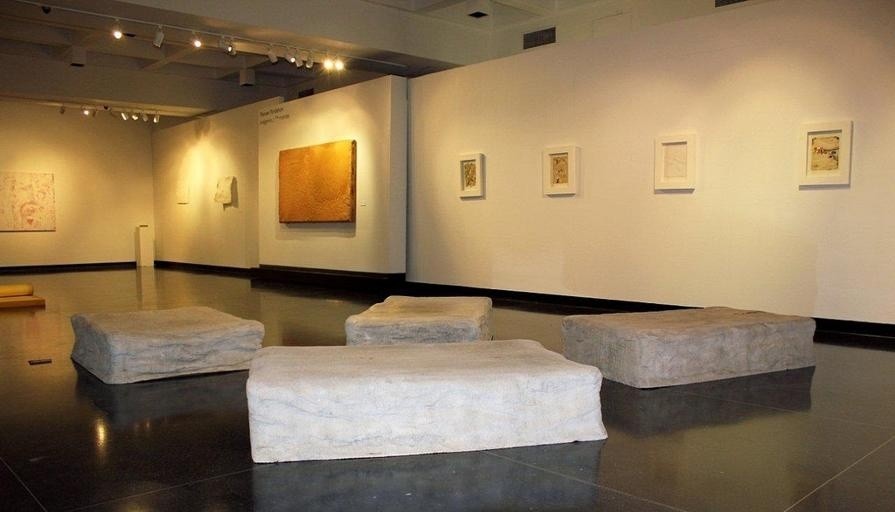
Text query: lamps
68 19 345 86
60 102 160 123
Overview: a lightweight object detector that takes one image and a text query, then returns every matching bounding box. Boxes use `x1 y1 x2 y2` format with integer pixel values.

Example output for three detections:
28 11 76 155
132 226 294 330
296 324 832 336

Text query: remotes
28 358 52 365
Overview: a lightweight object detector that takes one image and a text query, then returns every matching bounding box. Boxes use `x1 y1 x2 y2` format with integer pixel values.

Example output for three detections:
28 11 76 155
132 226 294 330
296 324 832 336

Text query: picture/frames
652 130 696 191
801 120 853 185
457 151 484 198
541 143 577 195
